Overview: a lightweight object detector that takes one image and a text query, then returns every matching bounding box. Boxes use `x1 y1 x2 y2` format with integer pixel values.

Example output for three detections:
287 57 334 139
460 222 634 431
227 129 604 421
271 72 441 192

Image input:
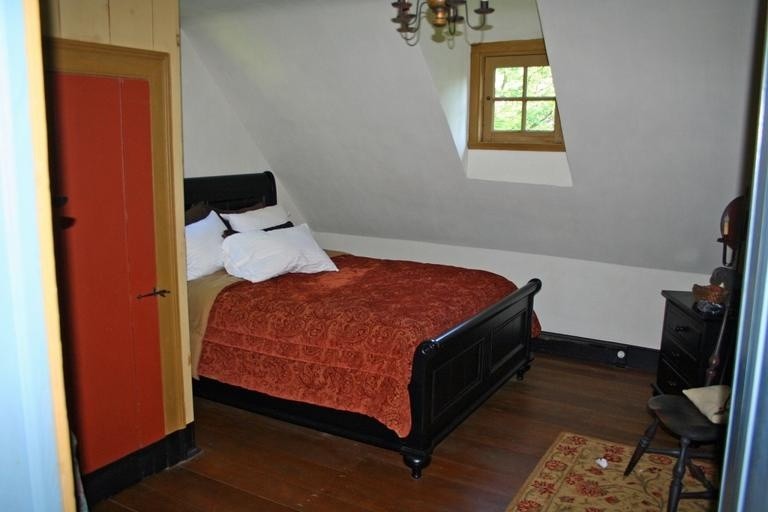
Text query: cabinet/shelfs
651 290 731 396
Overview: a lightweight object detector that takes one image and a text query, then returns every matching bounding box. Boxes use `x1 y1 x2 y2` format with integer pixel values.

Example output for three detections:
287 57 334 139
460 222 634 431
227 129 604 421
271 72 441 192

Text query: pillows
221 223 338 284
184 210 230 281
220 205 289 232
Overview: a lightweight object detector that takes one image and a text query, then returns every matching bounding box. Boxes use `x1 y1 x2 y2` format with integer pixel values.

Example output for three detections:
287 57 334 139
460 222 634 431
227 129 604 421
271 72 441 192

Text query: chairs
624 394 727 511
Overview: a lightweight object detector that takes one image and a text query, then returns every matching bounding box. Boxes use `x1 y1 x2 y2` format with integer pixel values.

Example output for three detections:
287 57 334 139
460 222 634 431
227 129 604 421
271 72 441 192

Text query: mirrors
720 195 745 249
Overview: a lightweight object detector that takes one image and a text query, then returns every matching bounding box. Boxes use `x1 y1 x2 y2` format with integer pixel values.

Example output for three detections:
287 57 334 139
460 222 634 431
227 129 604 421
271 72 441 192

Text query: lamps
391 0 495 36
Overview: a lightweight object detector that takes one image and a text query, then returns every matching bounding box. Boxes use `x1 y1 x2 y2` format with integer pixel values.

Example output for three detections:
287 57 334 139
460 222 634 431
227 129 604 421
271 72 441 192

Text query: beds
184 171 542 479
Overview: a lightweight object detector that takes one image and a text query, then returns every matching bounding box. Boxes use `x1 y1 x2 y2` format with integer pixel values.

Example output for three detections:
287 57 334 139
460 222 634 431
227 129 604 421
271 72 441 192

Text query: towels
683 385 731 424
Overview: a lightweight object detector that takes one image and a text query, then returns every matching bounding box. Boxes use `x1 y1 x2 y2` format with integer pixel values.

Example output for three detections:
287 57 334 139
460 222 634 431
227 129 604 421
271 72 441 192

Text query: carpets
506 431 717 512
